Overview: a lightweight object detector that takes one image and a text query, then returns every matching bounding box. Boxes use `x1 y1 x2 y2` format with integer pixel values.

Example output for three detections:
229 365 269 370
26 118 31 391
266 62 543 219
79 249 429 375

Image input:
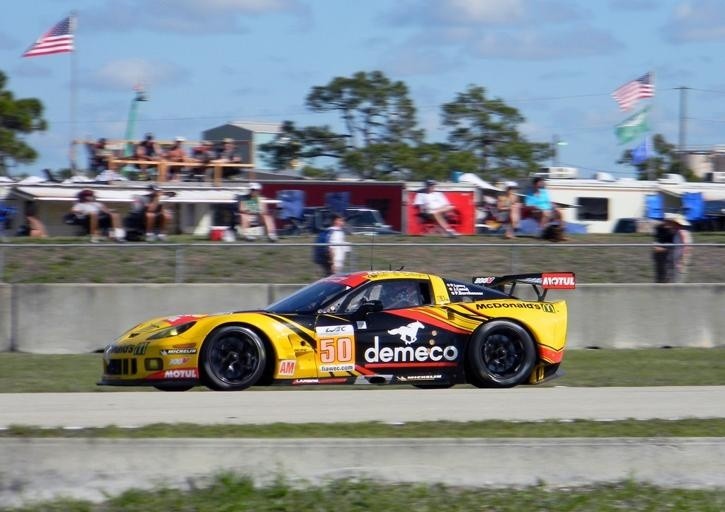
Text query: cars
276 206 401 237
94 268 577 393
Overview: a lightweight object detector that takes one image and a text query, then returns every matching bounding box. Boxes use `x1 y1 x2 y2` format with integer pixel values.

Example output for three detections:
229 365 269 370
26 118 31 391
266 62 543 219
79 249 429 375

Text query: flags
610 73 652 168
18 16 72 59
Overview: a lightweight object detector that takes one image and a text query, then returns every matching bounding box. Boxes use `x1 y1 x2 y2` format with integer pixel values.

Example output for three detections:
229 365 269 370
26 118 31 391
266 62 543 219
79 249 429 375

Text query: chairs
317 192 359 233
668 192 704 220
231 194 272 231
415 188 460 234
63 213 113 238
127 194 166 242
275 190 307 236
644 194 665 220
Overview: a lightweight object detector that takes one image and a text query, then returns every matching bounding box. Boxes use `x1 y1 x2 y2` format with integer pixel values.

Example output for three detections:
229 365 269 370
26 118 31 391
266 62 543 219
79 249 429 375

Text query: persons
94 135 243 180
413 180 461 238
16 206 48 239
497 185 523 238
68 189 127 244
526 177 571 240
237 181 278 240
322 213 351 280
654 211 697 282
131 182 178 241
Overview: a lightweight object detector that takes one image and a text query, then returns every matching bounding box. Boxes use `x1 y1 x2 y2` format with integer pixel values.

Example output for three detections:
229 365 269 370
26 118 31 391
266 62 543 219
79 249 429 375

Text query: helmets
384 285 422 306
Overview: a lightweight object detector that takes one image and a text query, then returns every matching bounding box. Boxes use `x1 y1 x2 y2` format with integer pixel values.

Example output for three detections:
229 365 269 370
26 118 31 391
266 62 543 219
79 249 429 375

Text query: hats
663 213 692 226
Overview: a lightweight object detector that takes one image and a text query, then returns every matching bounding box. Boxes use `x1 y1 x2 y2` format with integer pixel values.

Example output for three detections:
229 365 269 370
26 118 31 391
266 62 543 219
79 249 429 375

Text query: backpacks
313 229 342 265
